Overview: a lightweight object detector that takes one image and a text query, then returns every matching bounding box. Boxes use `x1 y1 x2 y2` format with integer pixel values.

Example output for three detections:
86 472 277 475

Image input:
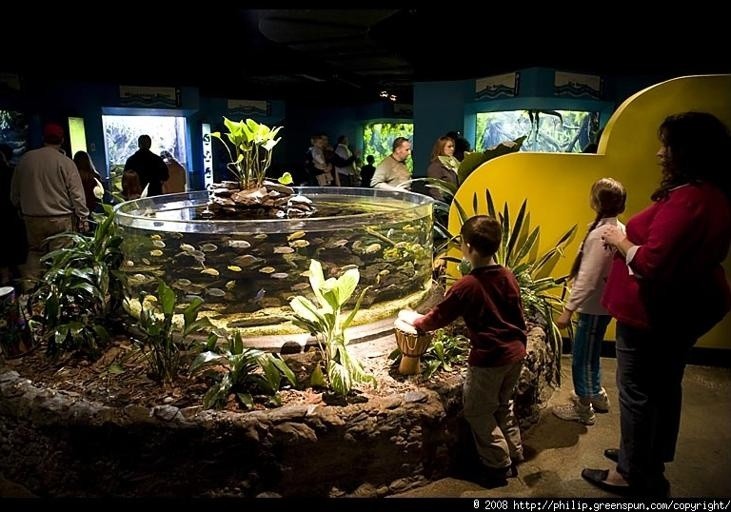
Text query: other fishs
121 221 434 324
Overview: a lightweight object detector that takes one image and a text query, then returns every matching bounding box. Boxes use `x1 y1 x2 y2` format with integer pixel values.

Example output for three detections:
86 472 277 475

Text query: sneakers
551 403 595 425
570 386 610 410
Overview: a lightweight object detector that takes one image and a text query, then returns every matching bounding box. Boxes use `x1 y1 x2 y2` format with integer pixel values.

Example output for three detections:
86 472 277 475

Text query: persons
551 177 626 426
0 122 187 295
303 131 471 241
582 111 731 498
397 215 527 486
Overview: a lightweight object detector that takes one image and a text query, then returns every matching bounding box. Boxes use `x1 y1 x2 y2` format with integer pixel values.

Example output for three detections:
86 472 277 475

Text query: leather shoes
603 448 618 462
581 467 630 494
